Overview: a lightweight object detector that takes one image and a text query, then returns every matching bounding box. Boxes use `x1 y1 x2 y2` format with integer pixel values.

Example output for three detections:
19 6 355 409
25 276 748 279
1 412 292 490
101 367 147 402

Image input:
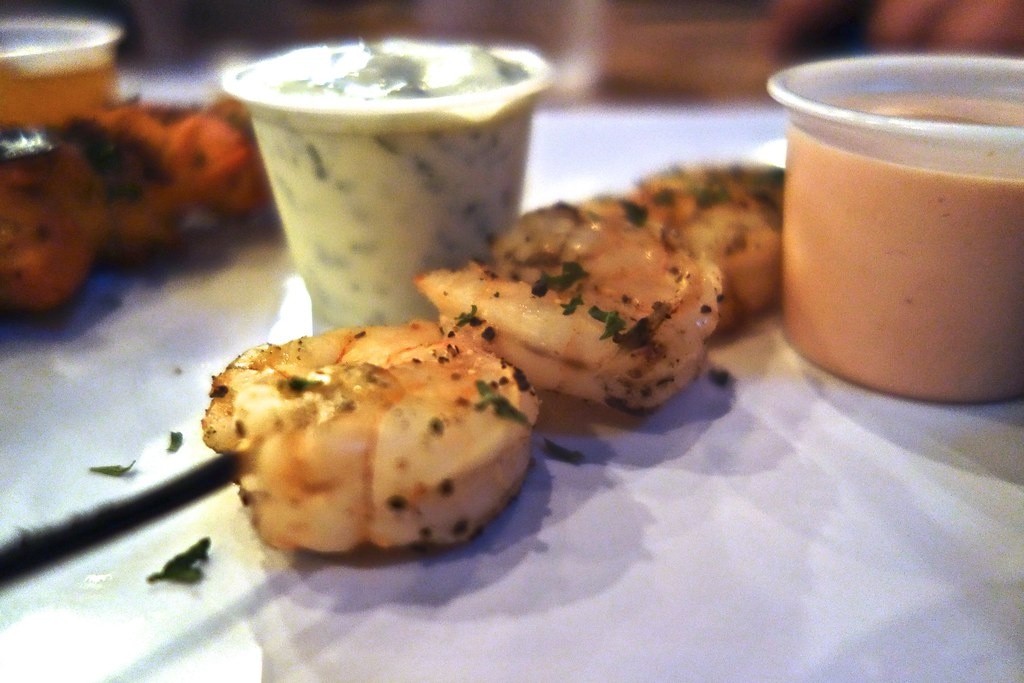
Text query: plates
0 81 1024 682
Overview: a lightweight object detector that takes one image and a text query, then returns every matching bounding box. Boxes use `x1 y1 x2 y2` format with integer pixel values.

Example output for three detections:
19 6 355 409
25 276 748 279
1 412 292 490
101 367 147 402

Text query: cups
0 16 125 129
227 39 551 323
766 51 1024 406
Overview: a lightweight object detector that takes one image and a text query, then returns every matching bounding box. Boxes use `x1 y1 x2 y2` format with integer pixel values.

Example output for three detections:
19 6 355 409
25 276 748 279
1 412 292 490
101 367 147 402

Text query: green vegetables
88 155 784 583
